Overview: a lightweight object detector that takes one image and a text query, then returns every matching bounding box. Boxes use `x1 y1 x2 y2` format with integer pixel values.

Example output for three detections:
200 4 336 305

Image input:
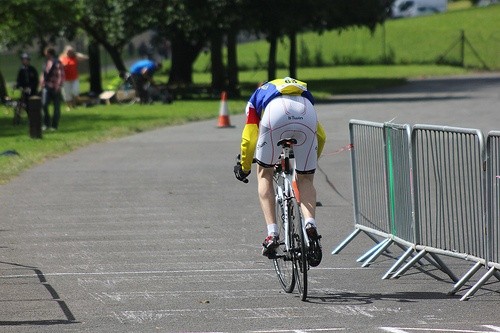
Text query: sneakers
262 232 279 256
304 221 322 267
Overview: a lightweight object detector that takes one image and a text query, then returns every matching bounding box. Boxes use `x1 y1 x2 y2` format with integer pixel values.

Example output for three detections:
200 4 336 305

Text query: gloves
233 165 252 184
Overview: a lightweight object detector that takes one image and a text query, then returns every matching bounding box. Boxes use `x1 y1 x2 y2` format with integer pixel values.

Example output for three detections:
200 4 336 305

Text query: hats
21 53 31 60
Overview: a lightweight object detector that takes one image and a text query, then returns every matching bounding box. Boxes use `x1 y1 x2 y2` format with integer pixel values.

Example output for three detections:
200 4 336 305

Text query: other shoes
41 125 47 131
67 107 71 111
49 127 57 131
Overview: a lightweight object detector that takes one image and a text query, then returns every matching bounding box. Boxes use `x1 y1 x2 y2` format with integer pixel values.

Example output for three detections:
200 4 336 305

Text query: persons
40 47 64 131
119 72 136 88
58 46 89 111
130 60 163 104
13 53 38 120
234 77 325 268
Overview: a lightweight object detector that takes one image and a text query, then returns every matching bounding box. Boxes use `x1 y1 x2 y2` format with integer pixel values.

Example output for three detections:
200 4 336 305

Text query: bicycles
234 137 323 302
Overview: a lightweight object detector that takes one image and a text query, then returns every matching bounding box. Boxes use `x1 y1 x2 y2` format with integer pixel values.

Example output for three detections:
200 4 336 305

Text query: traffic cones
216 92 236 129
